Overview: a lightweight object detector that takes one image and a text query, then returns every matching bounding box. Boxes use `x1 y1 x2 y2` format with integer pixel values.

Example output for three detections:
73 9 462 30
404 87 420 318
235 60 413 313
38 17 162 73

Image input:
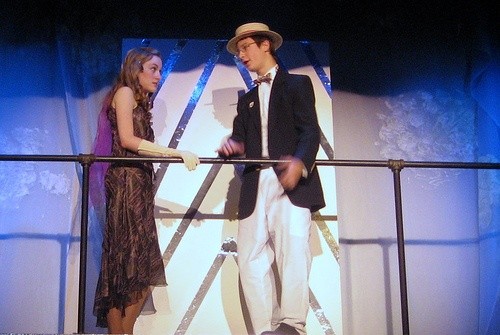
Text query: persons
92 47 202 335
214 22 326 335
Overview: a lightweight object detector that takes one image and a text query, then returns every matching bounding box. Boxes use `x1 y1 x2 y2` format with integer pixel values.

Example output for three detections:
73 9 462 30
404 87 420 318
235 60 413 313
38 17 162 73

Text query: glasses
235 43 256 55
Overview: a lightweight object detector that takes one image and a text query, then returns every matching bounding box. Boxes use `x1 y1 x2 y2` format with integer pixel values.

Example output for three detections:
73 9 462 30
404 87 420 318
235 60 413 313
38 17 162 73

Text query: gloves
138 139 200 171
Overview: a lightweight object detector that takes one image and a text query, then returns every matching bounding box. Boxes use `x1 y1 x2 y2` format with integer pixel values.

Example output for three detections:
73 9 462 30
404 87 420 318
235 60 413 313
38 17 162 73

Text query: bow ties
254 73 272 84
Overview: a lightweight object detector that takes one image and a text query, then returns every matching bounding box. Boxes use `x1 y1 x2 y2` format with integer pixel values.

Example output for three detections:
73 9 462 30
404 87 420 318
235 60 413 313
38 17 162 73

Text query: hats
227 22 283 55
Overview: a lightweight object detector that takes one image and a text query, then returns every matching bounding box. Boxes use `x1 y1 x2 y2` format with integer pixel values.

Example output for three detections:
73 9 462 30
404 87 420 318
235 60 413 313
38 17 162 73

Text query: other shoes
261 323 299 335
